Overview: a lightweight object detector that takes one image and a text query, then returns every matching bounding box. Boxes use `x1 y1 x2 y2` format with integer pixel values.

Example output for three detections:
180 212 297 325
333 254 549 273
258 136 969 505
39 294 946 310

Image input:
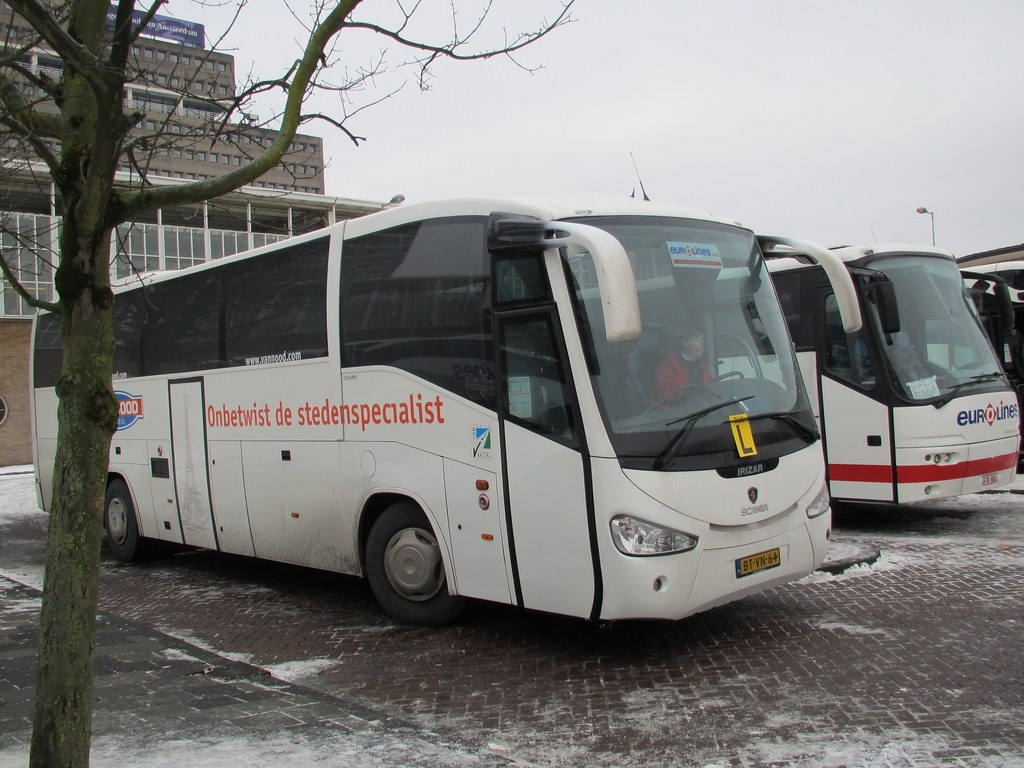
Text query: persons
651 329 713 410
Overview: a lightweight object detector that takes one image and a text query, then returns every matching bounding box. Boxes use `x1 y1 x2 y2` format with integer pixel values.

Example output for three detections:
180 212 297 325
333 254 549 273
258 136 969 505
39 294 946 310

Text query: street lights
916 206 936 247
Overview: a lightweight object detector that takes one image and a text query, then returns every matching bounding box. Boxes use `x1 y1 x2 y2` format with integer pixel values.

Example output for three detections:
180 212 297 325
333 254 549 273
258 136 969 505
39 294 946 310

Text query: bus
577 240 1022 506
28 193 864 630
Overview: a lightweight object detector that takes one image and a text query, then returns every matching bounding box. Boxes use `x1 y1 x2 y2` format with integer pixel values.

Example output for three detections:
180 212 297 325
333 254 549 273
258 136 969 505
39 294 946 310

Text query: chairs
628 330 663 408
